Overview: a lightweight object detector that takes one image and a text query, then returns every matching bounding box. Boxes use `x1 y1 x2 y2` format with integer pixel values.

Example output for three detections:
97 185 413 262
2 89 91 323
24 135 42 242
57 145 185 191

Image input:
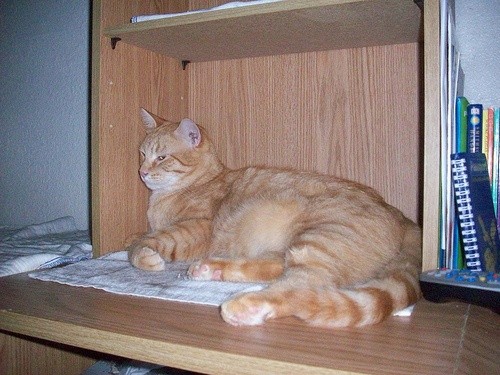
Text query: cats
129 105 421 328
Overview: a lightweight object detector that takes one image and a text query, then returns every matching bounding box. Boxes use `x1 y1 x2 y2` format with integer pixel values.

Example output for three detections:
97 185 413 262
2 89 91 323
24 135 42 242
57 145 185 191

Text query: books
440 1 499 272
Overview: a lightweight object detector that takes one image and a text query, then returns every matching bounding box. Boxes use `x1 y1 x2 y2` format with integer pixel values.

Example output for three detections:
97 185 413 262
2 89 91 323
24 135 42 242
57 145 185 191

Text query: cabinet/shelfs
92 0 441 272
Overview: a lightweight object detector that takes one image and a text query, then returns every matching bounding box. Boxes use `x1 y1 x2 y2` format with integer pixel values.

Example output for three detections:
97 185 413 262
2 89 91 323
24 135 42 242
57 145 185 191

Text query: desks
0 258 500 375
